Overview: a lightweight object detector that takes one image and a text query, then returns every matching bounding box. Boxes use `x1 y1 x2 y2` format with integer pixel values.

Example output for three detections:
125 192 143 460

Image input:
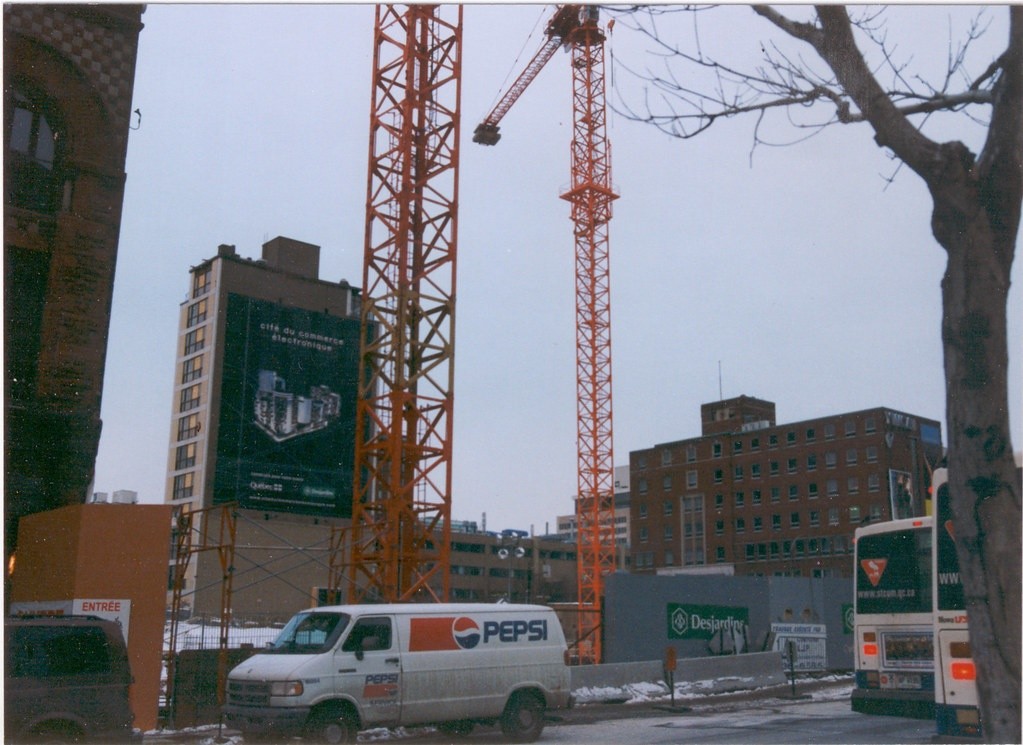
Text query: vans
218 603 575 745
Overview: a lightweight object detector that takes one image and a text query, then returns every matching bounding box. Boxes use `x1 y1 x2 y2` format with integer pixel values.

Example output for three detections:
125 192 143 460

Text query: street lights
496 546 525 601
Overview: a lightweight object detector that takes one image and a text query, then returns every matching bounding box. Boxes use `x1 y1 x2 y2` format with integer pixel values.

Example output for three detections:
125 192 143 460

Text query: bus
850 514 936 715
932 452 1023 743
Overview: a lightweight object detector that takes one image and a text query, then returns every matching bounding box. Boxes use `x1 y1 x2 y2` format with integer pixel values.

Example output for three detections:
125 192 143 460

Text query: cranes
472 2 619 664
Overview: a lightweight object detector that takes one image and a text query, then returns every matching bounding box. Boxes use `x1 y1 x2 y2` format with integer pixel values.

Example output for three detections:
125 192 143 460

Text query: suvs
4 614 145 745
